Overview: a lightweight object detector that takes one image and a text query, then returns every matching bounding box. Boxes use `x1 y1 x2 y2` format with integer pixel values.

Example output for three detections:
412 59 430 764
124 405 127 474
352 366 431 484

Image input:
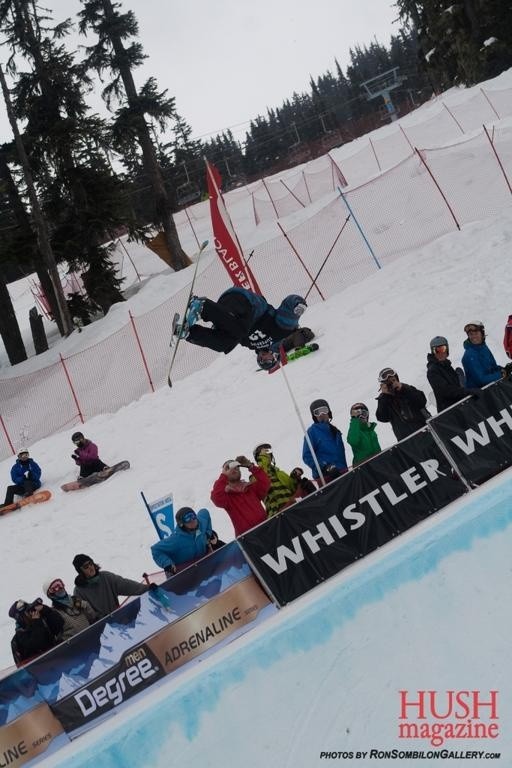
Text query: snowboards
0 491 51 518
60 460 130 492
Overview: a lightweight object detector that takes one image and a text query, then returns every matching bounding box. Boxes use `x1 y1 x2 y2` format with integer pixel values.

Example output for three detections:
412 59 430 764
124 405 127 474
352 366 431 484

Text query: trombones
167 241 208 388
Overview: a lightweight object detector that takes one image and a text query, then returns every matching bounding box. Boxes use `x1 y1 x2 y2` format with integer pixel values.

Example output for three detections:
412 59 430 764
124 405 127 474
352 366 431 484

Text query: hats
310 399 333 423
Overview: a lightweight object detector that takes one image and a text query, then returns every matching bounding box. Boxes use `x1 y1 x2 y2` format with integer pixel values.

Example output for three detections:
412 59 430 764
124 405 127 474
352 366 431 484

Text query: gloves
163 563 177 575
234 456 253 469
323 463 341 480
72 449 80 460
23 471 32 480
148 583 161 590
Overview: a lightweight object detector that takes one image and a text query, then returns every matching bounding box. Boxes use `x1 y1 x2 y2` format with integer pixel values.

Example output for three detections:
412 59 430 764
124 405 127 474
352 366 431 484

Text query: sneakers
0 491 34 508
176 320 189 341
188 297 205 328
77 465 111 480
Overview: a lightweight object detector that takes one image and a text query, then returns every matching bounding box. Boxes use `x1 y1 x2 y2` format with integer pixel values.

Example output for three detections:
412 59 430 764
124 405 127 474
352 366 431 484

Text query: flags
207 162 262 297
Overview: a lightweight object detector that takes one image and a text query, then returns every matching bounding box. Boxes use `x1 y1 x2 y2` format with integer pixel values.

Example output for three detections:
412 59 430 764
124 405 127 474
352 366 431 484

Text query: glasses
313 405 330 416
182 512 198 524
80 561 94 569
379 368 396 382
48 581 64 595
258 356 276 369
465 324 485 333
431 345 447 354
355 408 367 417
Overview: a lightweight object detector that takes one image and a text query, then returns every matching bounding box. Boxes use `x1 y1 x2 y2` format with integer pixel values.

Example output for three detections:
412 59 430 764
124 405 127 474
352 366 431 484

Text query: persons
347 402 381 469
0 447 42 508
461 320 507 392
426 336 481 413
71 432 110 481
374 368 433 443
303 398 348 489
249 443 304 520
7 454 279 676
503 314 512 360
174 287 315 370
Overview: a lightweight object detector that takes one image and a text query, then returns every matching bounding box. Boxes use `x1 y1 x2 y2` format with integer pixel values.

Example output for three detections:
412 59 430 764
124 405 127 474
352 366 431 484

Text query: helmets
429 336 449 355
175 506 196 527
43 572 65 598
252 440 273 458
9 600 29 622
256 351 282 370
350 403 368 417
72 553 92 573
16 448 30 458
379 368 399 385
464 319 485 336
71 432 84 443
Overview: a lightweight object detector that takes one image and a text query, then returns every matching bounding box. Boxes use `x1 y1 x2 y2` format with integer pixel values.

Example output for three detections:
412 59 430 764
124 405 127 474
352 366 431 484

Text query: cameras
229 461 241 470
25 598 43 614
384 379 399 393
